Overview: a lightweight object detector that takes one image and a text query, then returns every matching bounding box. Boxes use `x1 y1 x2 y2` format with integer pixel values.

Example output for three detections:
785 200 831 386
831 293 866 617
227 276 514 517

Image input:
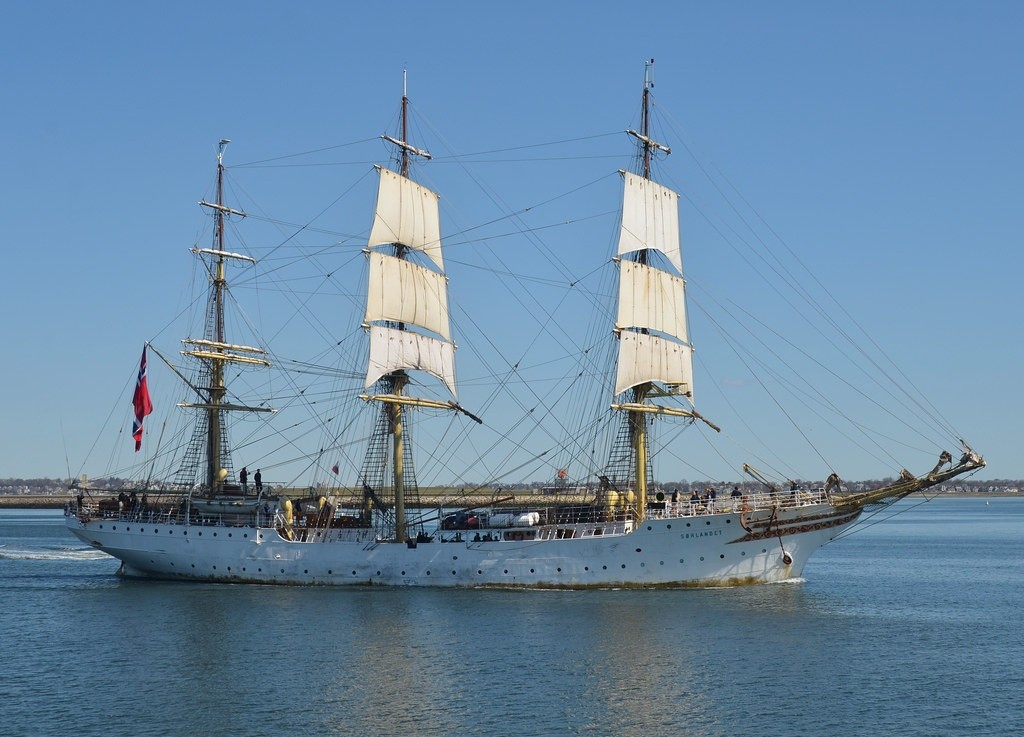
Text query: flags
132 347 153 452
332 462 340 474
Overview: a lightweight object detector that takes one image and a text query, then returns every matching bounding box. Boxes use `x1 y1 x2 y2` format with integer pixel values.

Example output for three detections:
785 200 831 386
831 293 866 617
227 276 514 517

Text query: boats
191 495 281 517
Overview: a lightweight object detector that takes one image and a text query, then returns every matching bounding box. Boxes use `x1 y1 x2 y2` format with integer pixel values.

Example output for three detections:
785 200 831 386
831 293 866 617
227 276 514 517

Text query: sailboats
63 58 986 590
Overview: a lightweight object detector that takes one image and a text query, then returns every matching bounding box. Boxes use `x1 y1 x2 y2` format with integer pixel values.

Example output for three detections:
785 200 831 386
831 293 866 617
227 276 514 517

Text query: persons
240 467 247 495
731 487 742 496
77 495 84 511
790 483 798 494
254 469 265 495
670 489 681 513
691 487 716 508
295 499 301 527
119 491 148 514
